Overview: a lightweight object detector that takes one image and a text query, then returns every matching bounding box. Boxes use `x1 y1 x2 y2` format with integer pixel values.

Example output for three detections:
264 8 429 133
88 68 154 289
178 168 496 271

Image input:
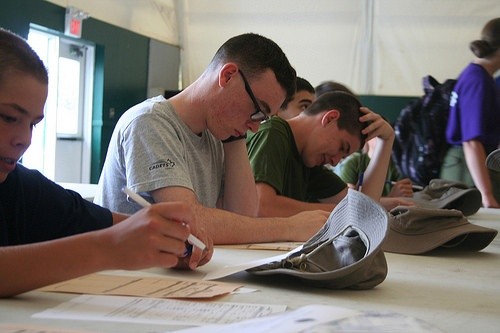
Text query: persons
248 92 396 217
275 76 413 212
441 18 500 208
92 32 331 245
0 27 214 298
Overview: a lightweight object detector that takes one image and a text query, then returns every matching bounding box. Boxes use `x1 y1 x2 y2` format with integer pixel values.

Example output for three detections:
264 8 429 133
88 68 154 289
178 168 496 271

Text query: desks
0 205 500 332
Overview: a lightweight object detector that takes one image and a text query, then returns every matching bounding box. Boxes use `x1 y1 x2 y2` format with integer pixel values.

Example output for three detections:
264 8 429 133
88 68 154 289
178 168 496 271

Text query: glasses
239 69 271 125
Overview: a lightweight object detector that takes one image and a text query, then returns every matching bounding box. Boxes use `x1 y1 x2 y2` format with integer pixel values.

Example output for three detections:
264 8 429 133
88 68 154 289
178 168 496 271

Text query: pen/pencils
121 187 210 253
391 181 423 189
357 174 363 192
358 185 363 192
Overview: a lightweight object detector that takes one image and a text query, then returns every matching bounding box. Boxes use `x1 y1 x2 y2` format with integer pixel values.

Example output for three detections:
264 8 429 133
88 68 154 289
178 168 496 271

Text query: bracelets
221 132 247 143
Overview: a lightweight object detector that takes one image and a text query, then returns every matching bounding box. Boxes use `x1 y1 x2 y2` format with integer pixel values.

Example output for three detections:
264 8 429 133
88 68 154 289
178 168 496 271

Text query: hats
414 179 482 215
485 145 500 173
245 188 388 291
382 205 498 255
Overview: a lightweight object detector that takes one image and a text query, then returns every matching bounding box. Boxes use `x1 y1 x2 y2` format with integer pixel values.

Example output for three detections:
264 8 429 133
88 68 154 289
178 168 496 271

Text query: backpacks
392 75 458 186
